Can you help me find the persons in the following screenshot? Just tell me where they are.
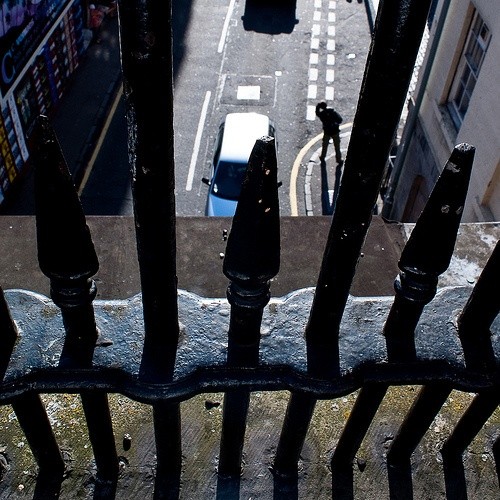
[94,5,117,62]
[316,101,343,164]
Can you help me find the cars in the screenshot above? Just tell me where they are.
[199,112,284,217]
